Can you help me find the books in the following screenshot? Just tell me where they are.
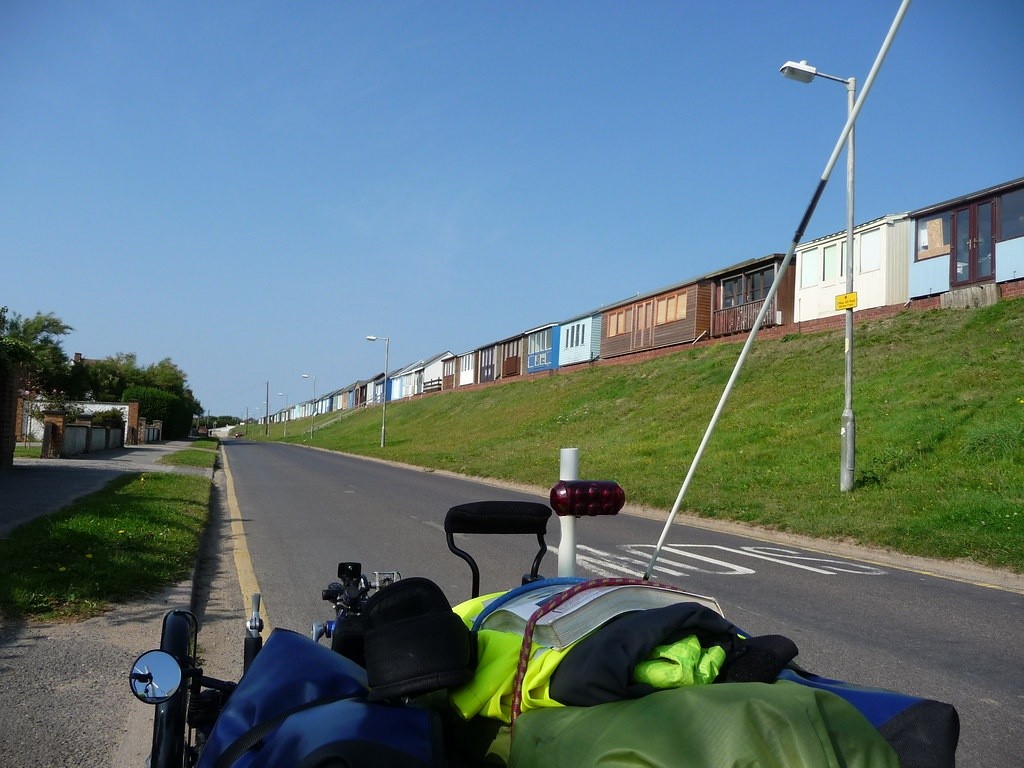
[468,584,725,651]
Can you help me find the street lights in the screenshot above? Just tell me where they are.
[779,58,859,490]
[277,392,288,437]
[365,335,390,448]
[255,407,263,436]
[302,374,316,439]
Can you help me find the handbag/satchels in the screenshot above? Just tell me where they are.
[198,627,430,768]
[361,577,468,700]
